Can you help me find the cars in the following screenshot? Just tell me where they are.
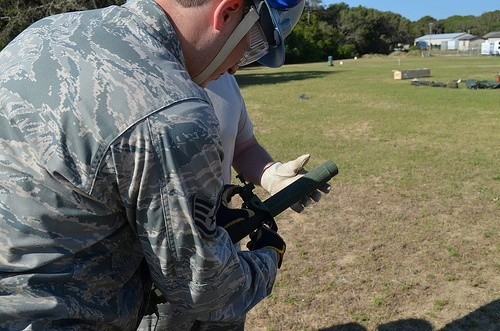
[393,46,405,51]
[488,81,500,89]
[432,82,447,88]
[403,44,411,51]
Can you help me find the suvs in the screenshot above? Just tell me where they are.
[466,79,478,89]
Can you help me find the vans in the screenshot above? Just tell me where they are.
[476,80,492,89]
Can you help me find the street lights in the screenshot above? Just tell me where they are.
[428,22,434,56]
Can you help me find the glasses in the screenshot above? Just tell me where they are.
[237,21,269,67]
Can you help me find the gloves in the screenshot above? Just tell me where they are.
[216,184,255,230]
[260,154,331,213]
[247,225,287,269]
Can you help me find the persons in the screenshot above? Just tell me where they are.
[137,70,331,331]
[0,0,305,331]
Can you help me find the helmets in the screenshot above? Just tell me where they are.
[250,0,305,69]
